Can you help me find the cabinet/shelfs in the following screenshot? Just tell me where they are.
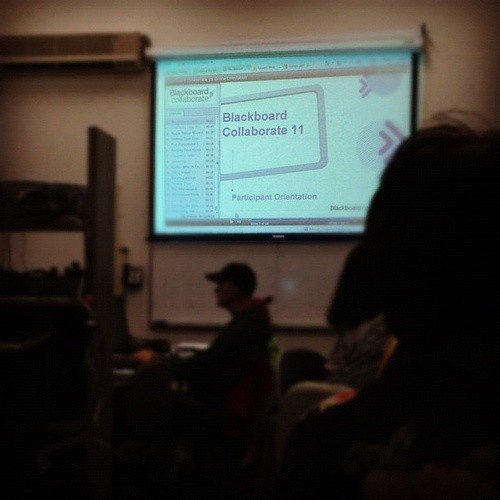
[0,122,117,500]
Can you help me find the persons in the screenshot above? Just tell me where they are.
[280,109,500,500]
[133,260,273,439]
[281,314,387,415]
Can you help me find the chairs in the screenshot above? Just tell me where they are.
[275,382,343,439]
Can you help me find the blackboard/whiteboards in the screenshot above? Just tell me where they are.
[147,234,360,337]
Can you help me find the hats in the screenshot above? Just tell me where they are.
[205,260,257,297]
